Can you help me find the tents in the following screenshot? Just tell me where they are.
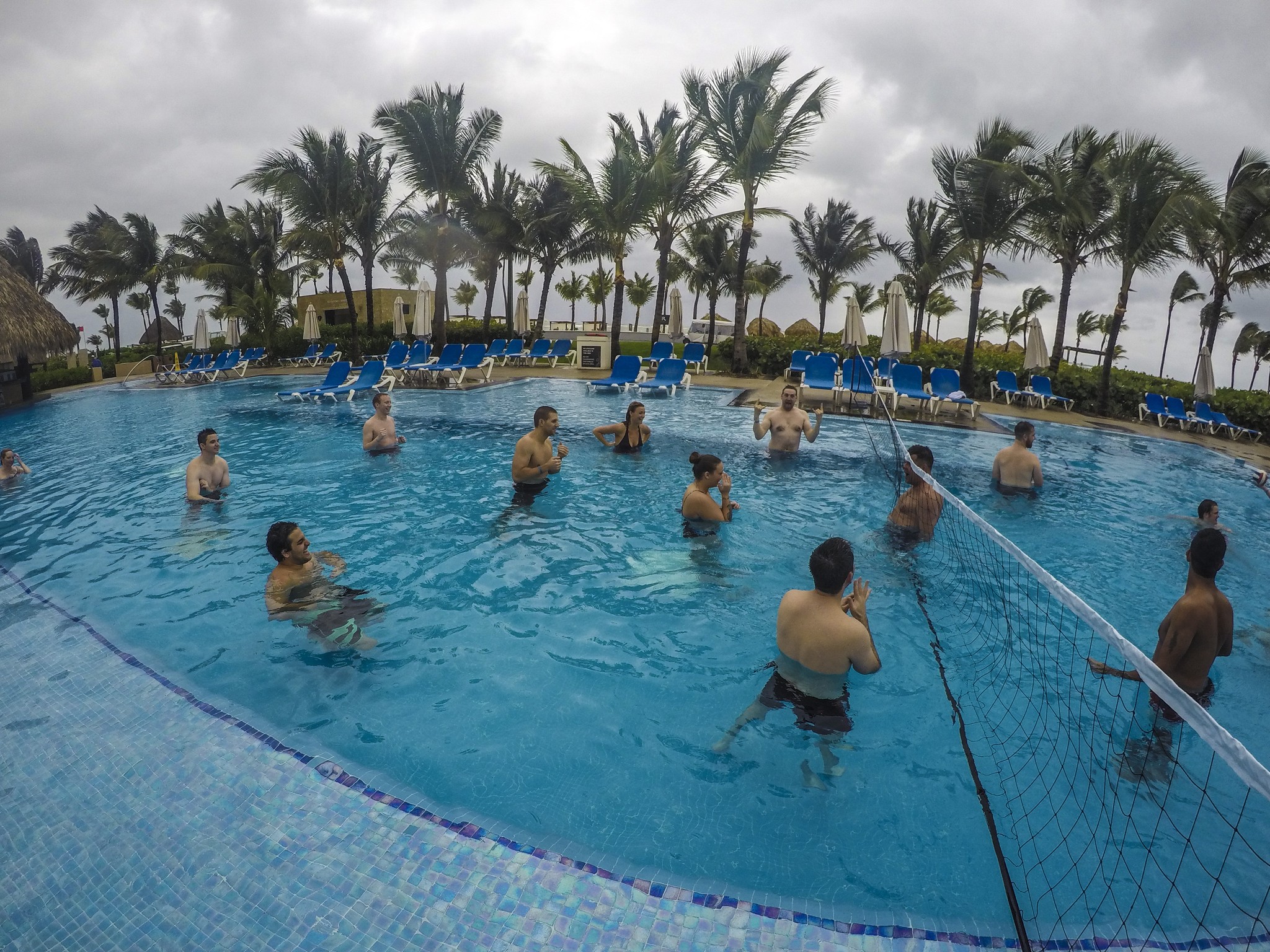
[701,312,1021,351]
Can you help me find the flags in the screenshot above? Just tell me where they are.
[77,326,83,332]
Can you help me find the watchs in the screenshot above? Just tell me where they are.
[753,419,759,423]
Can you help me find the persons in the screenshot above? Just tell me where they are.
[1088,499,1235,782]
[753,384,824,453]
[885,446,944,545]
[186,428,231,502]
[362,393,407,451]
[511,401,652,494]
[680,452,741,538]
[0,449,33,482]
[989,420,1047,488]
[1252,481,1270,498]
[265,520,378,652]
[712,538,881,754]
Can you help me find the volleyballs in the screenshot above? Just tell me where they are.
[1253,470,1267,486]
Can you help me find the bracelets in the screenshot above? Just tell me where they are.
[538,465,544,478]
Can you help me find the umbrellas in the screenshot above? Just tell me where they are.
[0,256,82,401]
[139,316,183,345]
[514,287,531,350]
[1193,346,1216,433]
[840,276,912,387]
[303,302,321,356]
[191,308,212,381]
[224,316,241,352]
[1021,312,1051,393]
[392,276,432,343]
[667,285,683,359]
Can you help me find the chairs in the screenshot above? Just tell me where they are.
[583,340,709,399]
[153,347,268,384]
[783,349,982,422]
[1138,391,1263,448]
[274,338,577,405]
[990,370,1076,414]
